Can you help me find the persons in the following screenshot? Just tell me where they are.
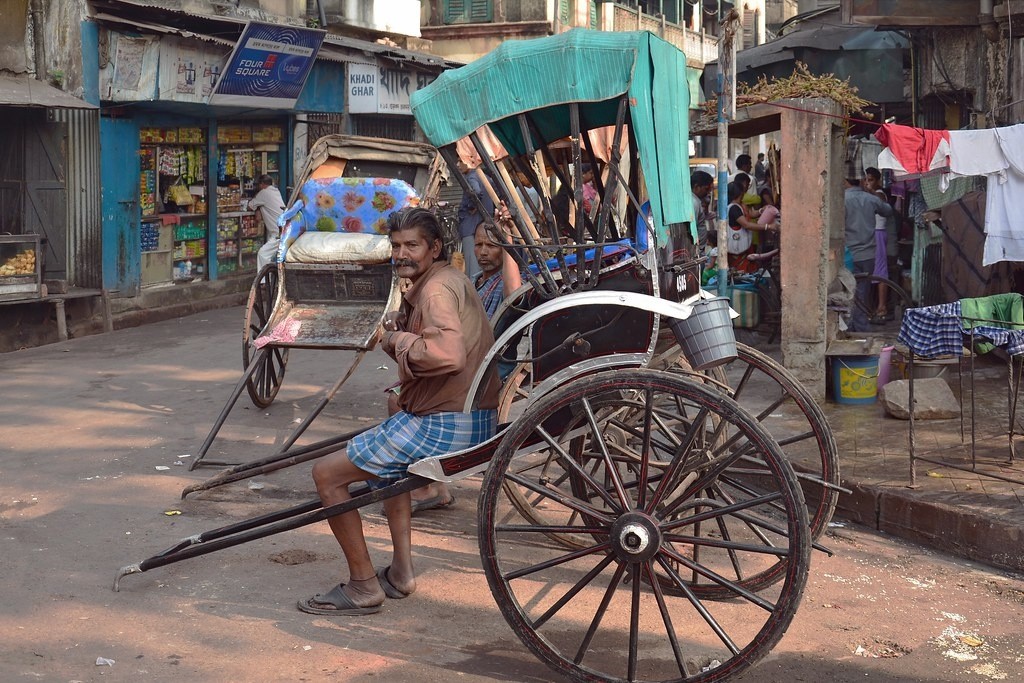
[247,175,286,291]
[379,153,902,516]
[297,208,506,615]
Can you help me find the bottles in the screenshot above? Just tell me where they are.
[173,242,187,259]
[178,259,192,277]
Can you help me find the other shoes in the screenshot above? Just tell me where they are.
[868,316,885,324]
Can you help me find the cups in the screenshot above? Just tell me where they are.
[757,205,779,225]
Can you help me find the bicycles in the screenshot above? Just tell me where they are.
[705,248,916,352]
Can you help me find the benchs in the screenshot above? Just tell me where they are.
[275,176,420,264]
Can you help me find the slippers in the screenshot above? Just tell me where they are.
[295,583,383,615]
[373,564,406,599]
[430,494,455,509]
[382,494,442,517]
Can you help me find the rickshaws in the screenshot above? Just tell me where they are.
[113,29,856,683]
[186,132,452,476]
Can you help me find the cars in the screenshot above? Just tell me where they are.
[688,157,735,199]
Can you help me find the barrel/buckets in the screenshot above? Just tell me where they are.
[664,269,739,371]
[856,339,895,393]
[912,364,951,385]
[828,354,880,405]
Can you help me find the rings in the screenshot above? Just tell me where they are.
[385,321,388,324]
[388,320,391,324]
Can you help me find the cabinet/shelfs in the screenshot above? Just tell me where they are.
[139,211,266,290]
